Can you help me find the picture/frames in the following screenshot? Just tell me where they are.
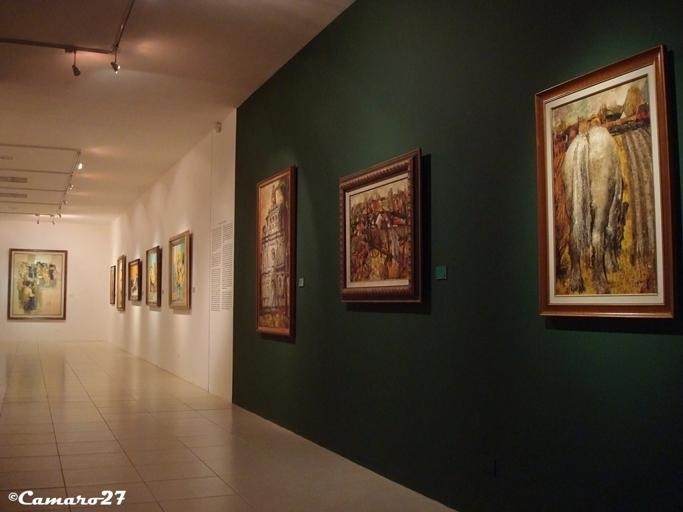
[7,248,68,321]
[255,165,297,337]
[167,229,193,310]
[534,44,676,322]
[115,254,126,310]
[337,146,425,306]
[109,266,116,305]
[146,246,162,307]
[128,258,142,301]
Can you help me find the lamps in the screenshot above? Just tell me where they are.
[109,52,121,75]
[69,51,82,80]
